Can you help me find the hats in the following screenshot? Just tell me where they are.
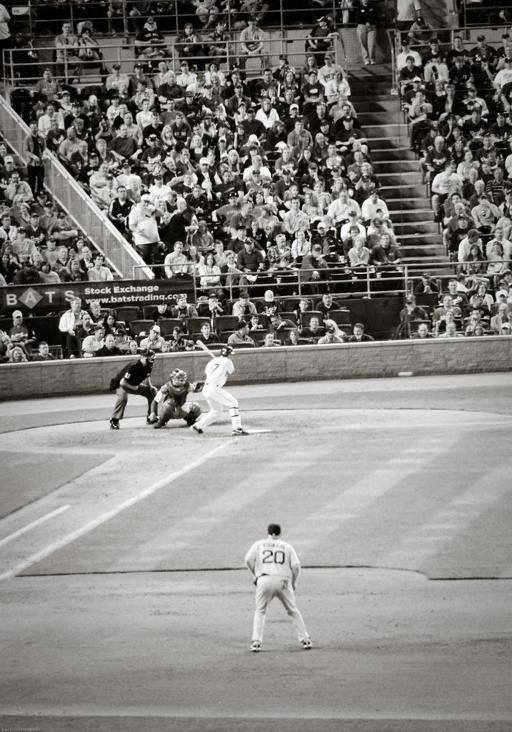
[497,280,508,286]
[423,272,431,278]
[207,289,274,303]
[151,326,161,334]
[269,525,280,536]
[405,294,416,304]
[401,34,509,265]
[13,310,22,318]
[5,7,390,269]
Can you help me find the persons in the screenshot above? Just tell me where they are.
[244,524,312,652]
[110,348,157,429]
[150,368,204,428]
[191,345,250,436]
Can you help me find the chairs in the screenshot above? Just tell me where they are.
[17,287,500,358]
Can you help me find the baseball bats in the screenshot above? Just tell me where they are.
[196,339,215,358]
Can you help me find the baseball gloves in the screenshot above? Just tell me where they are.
[192,381,204,392]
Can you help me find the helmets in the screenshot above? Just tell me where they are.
[170,368,186,392]
[221,346,235,356]
[140,348,155,369]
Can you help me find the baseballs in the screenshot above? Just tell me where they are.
[398,371,413,377]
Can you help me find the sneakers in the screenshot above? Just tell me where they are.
[303,640,312,649]
[154,421,166,428]
[147,415,158,424]
[111,421,120,429]
[187,421,197,427]
[232,429,248,435]
[191,425,203,433]
[249,643,261,651]
